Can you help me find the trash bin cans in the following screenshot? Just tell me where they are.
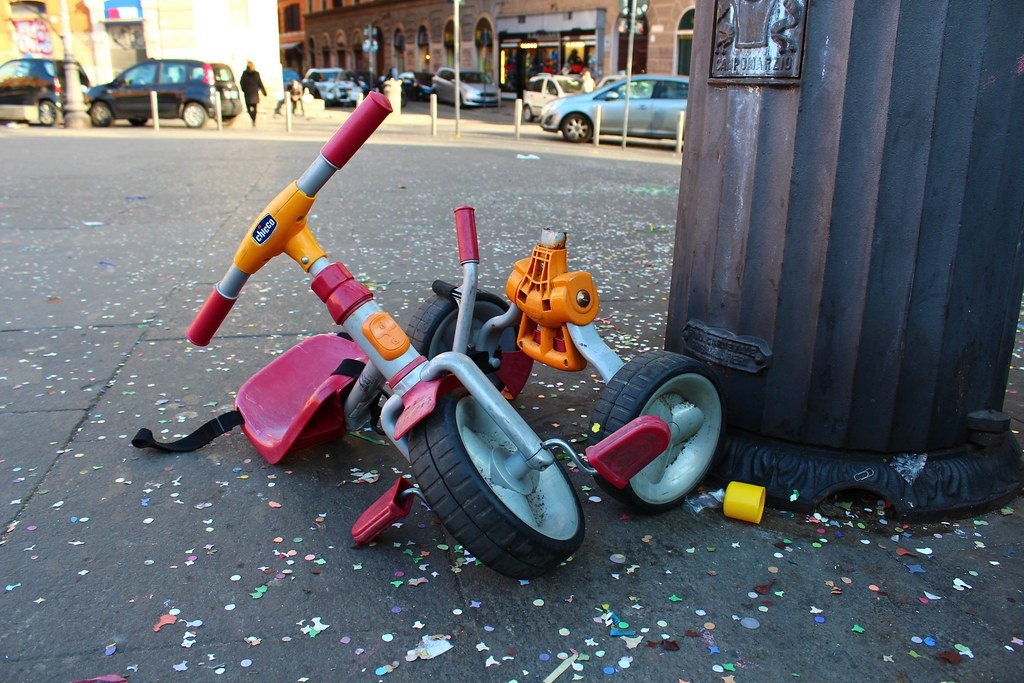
[659,1,1023,518]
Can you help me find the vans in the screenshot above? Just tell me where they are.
[432,67,499,106]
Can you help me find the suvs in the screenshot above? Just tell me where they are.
[83,58,242,129]
[521,72,584,122]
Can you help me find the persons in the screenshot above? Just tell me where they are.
[287,78,307,117]
[240,60,266,124]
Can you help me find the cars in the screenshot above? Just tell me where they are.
[282,69,300,89]
[0,57,94,128]
[332,72,405,111]
[539,75,689,144]
[303,67,348,102]
[400,72,438,101]
